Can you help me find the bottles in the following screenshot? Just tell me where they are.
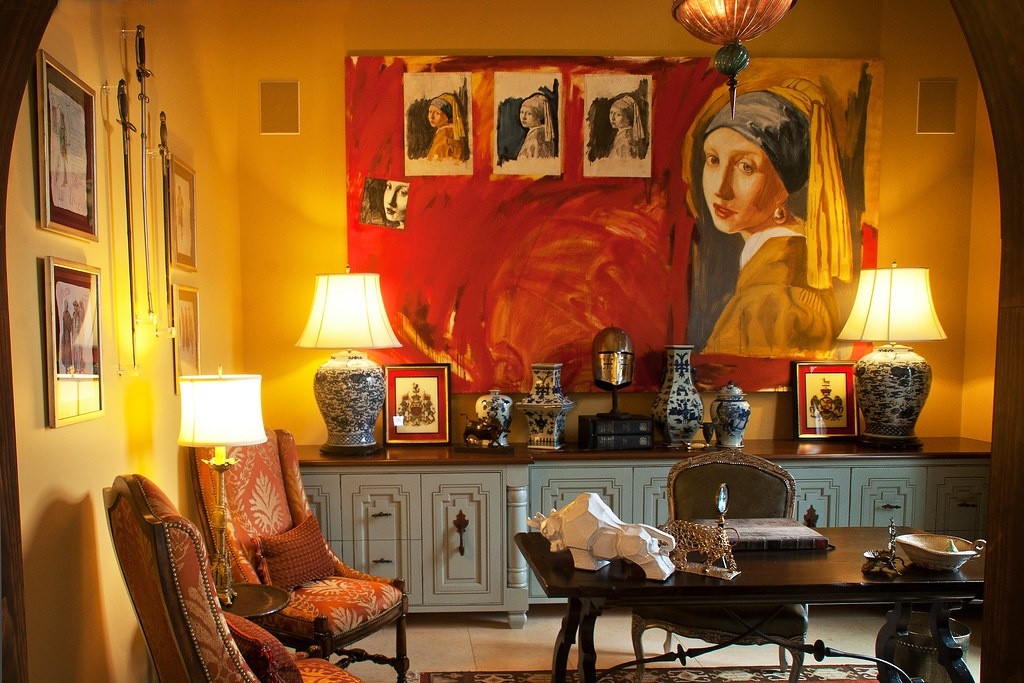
[652,344,704,450]
[710,381,751,448]
[475,390,513,447]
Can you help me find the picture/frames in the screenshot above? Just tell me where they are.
[35,50,200,429]
[382,365,453,449]
[792,361,859,443]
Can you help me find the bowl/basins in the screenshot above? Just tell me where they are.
[894,533,986,571]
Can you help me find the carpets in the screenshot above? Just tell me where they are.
[420,664,885,683]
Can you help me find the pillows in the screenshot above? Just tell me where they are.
[251,516,337,590]
[219,610,305,683]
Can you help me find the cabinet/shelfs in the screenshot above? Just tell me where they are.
[300,459,993,628]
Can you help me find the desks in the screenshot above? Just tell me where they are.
[216,584,292,618]
[513,525,985,683]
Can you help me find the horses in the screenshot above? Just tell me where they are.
[463,419,511,449]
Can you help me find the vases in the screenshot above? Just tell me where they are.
[476,362,574,450]
[653,345,704,449]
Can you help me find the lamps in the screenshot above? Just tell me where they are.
[178,375,268,605]
[672,0,799,118]
[835,268,948,451]
[296,274,403,456]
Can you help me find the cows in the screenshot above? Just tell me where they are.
[654,519,741,575]
[525,492,676,583]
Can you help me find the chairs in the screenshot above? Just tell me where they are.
[103,429,409,683]
[632,450,808,682]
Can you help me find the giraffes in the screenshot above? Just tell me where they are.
[861,518,903,576]
